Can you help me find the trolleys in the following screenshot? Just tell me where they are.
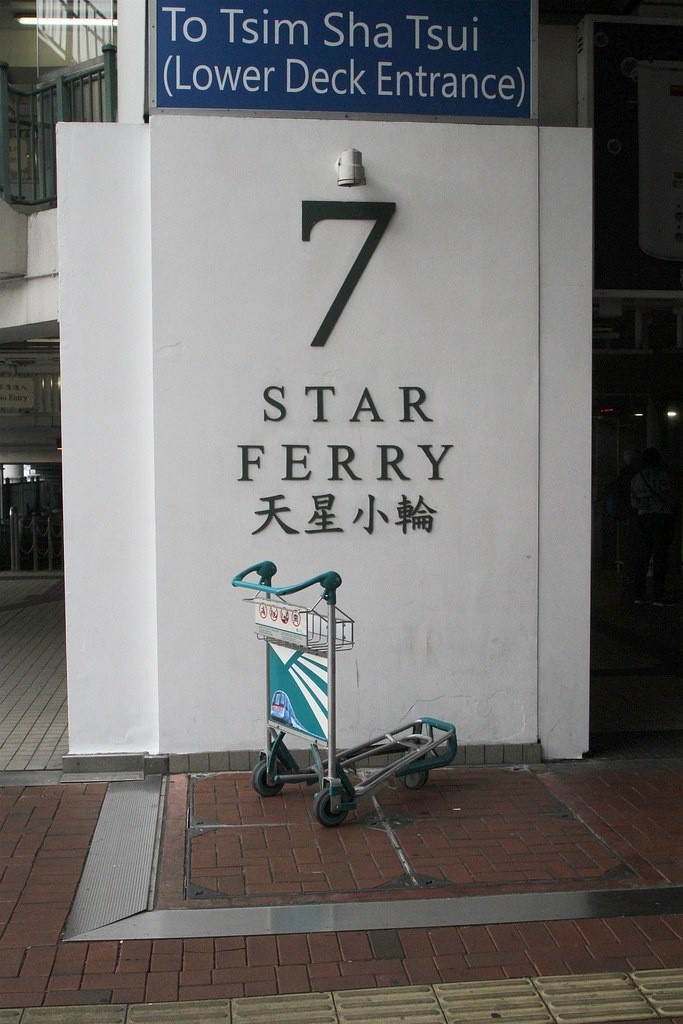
[231,560,457,828]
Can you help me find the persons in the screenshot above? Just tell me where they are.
[618,445,642,598]
[628,449,676,607]
